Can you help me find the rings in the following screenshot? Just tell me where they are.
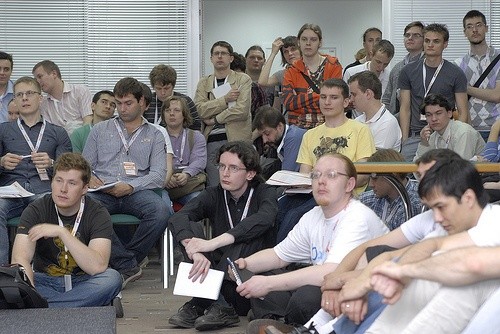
[346,305,350,308]
[325,302,329,304]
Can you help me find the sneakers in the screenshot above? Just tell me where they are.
[169,297,205,328]
[264,320,320,334]
[195,303,240,330]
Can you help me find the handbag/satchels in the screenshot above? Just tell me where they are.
[168,168,207,199]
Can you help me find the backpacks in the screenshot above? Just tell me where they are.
[0,263,48,309]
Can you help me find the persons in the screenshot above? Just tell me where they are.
[10,153,124,318]
[227,151,390,325]
[246,148,500,334]
[167,141,278,330]
[0,10,500,287]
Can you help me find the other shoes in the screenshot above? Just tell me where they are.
[137,256,150,269]
[120,265,143,289]
[110,298,123,318]
[246,320,296,334]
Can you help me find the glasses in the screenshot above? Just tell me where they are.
[310,171,349,181]
[464,23,486,29]
[213,51,231,57]
[14,90,41,98]
[217,163,247,173]
[403,33,424,39]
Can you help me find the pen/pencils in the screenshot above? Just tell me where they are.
[426,123,430,143]
[20,154,31,158]
[93,171,105,186]
[227,258,235,267]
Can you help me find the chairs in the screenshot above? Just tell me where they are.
[73,127,175,292]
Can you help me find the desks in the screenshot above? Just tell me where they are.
[355,163,500,173]
